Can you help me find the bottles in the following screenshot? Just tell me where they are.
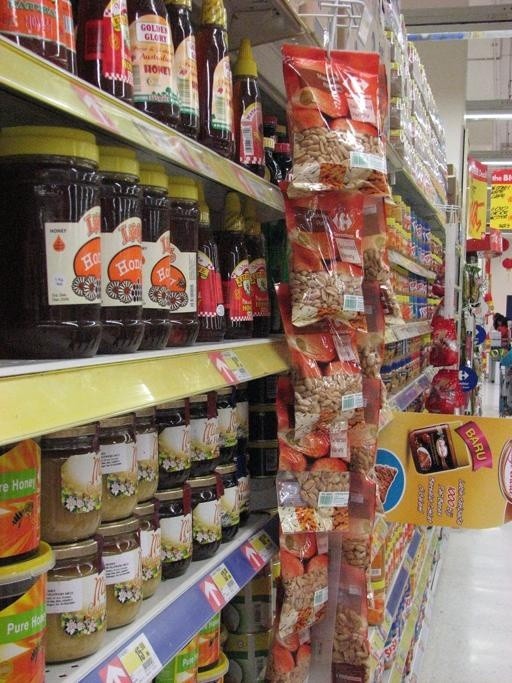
[1,1,289,186]
[195,180,272,343]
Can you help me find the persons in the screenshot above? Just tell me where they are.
[493,313,508,330]
[496,315,508,338]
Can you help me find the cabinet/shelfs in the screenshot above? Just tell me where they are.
[0,0,486,683]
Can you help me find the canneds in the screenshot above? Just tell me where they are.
[0,126,278,683]
